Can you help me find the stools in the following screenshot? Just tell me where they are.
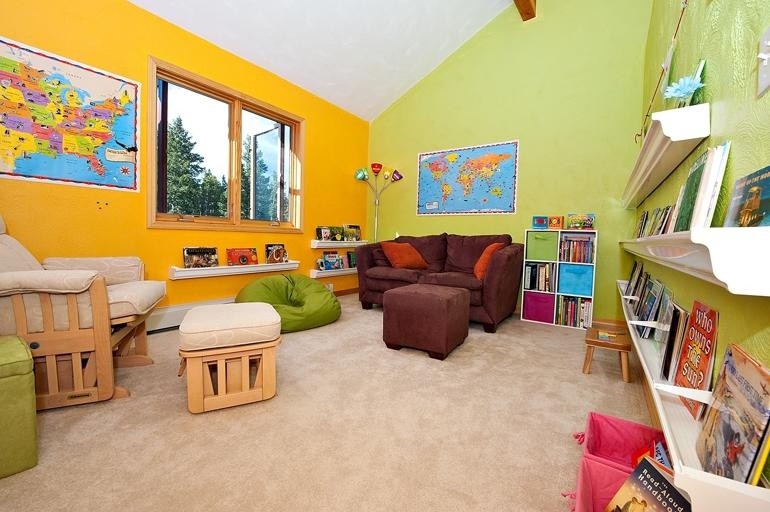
[582,318,632,384]
[0,333,39,481]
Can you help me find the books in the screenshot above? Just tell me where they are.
[525,261,557,292]
[652,286,719,422]
[315,223,362,270]
[623,259,664,339]
[695,342,770,485]
[605,439,692,512]
[723,165,770,227]
[669,140,731,232]
[631,204,675,239]
[559,236,594,263]
[554,293,591,331]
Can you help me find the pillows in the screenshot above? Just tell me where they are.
[472,242,505,282]
[379,241,430,271]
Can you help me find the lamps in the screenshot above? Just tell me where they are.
[354,159,404,243]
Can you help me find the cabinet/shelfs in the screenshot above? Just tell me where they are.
[615,100,770,511]
[306,236,359,279]
[520,228,599,331]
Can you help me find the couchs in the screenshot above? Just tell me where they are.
[235,273,342,335]
[382,282,472,361]
[177,300,282,417]
[0,209,168,413]
[354,231,524,334]
